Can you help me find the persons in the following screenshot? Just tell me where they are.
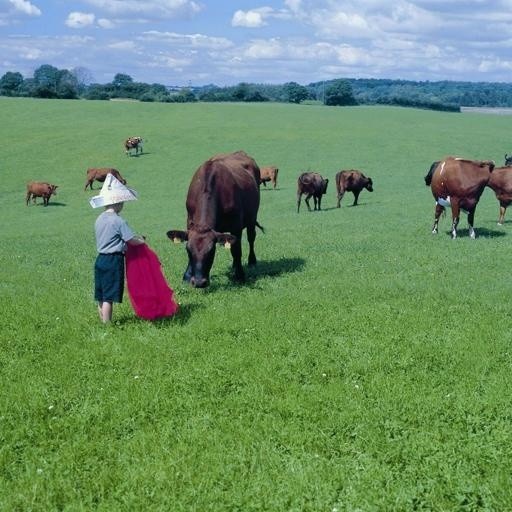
[90,172,138,324]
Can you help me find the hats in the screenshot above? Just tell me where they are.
[90,173,138,209]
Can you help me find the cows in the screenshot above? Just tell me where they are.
[334,169,374,208]
[83,167,128,192]
[125,136,144,156]
[25,181,59,207]
[259,165,279,190]
[424,155,496,241]
[295,171,329,214]
[485,164,512,226]
[165,151,266,289]
